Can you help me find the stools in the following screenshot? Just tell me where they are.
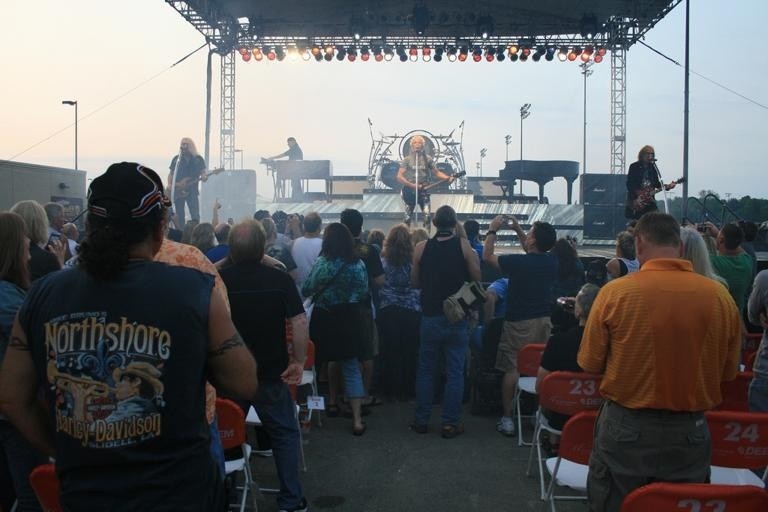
[493,181,516,204]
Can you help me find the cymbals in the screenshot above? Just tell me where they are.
[432,136,450,138]
[385,136,403,137]
[445,143,460,146]
[379,154,392,156]
[375,141,390,144]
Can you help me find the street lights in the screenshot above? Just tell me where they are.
[516,102,534,203]
[475,161,480,177]
[480,148,487,178]
[579,58,594,173]
[503,134,512,162]
[60,97,80,174]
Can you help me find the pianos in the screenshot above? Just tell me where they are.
[499,160,579,205]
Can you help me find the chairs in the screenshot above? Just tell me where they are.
[10,333,323,512]
[510,333,768,512]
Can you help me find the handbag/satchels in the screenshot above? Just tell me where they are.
[443,281,486,324]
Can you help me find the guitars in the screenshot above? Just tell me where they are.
[632,176,686,211]
[402,170,466,205]
[166,166,225,204]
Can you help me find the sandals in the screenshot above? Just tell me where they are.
[325,395,382,436]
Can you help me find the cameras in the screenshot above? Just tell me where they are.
[500,216,509,227]
[557,297,575,310]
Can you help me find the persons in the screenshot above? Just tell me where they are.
[267,136,308,198]
[623,145,677,220]
[538,211,768,512]
[397,136,456,229]
[1,203,86,511]
[378,205,584,439]
[164,137,208,232]
[164,205,387,512]
[0,161,260,505]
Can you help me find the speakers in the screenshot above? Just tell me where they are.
[583,173,628,206]
[584,206,626,240]
[201,170,257,229]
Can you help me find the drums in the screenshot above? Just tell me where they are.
[399,130,439,167]
[433,163,454,186]
[381,163,404,187]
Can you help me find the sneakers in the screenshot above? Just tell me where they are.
[440,424,460,437]
[280,497,308,512]
[409,422,428,433]
[496,417,514,436]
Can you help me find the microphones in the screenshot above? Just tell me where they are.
[415,146,419,152]
[648,158,657,161]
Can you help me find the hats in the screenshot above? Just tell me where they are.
[88,163,171,226]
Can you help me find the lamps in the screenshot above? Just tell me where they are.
[237,0,606,63]
